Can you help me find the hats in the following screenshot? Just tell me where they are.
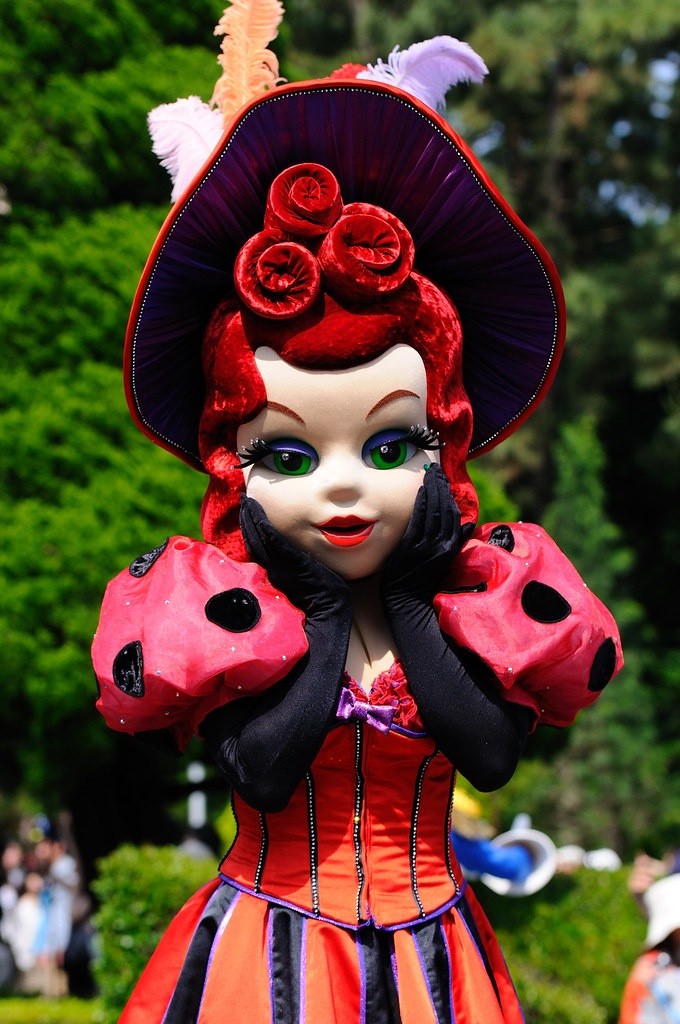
[642,873,680,950]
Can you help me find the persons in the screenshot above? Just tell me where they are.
[0,823,100,1001]
[618,822,680,1024]
[90,77,626,1024]
